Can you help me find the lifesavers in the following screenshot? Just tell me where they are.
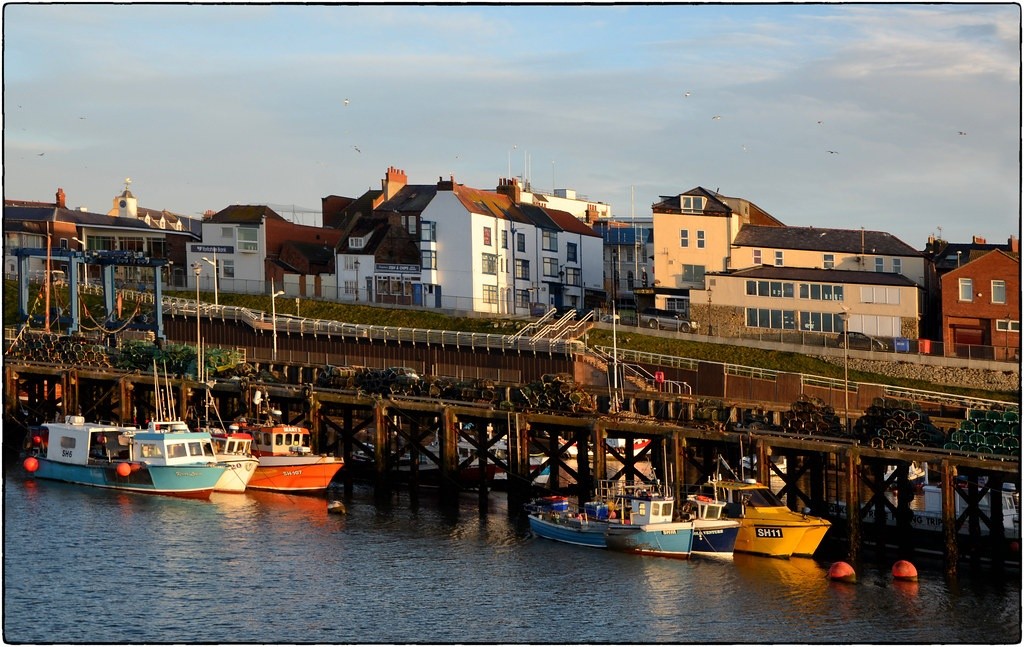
[695,496,712,502]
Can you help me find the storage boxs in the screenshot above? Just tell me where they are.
[585,502,608,519]
[543,496,569,510]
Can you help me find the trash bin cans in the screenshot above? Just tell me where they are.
[893,337,909,353]
[917,338,931,355]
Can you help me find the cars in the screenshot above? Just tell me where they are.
[836,331,888,352]
[600,314,617,324]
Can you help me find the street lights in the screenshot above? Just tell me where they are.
[706,286,714,336]
[201,247,218,314]
[270,276,285,361]
[837,312,852,438]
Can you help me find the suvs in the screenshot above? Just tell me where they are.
[638,307,702,333]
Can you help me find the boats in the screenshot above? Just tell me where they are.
[191,367,345,495]
[18,357,229,501]
[523,477,742,563]
[352,416,555,496]
[676,471,833,557]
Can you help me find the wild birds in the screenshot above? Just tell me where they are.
[956,131,966,135]
[354,146,361,153]
[828,151,838,154]
[684,91,693,97]
[344,99,349,106]
[818,121,823,124]
[36,152,44,156]
[711,115,721,120]
